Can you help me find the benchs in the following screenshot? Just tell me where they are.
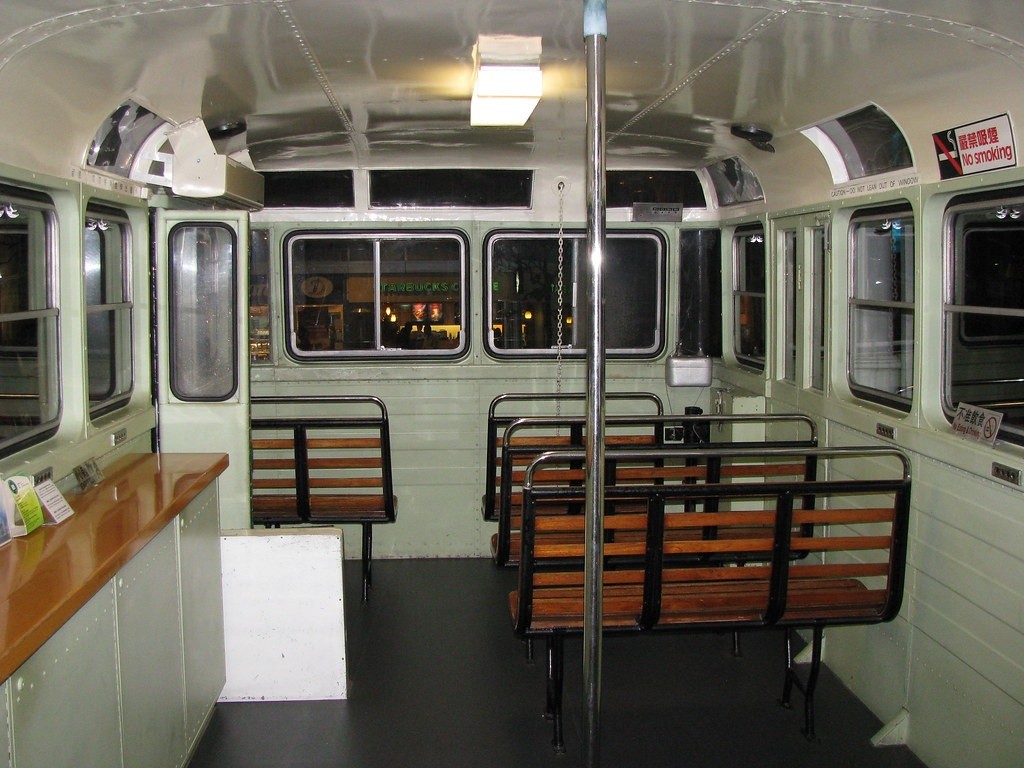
[480,391,663,521]
[489,413,817,663]
[249,395,397,603]
[509,445,912,757]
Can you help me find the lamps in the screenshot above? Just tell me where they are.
[471,33,542,71]
[470,66,543,126]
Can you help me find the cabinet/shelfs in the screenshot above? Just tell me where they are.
[0,453,229,768]
